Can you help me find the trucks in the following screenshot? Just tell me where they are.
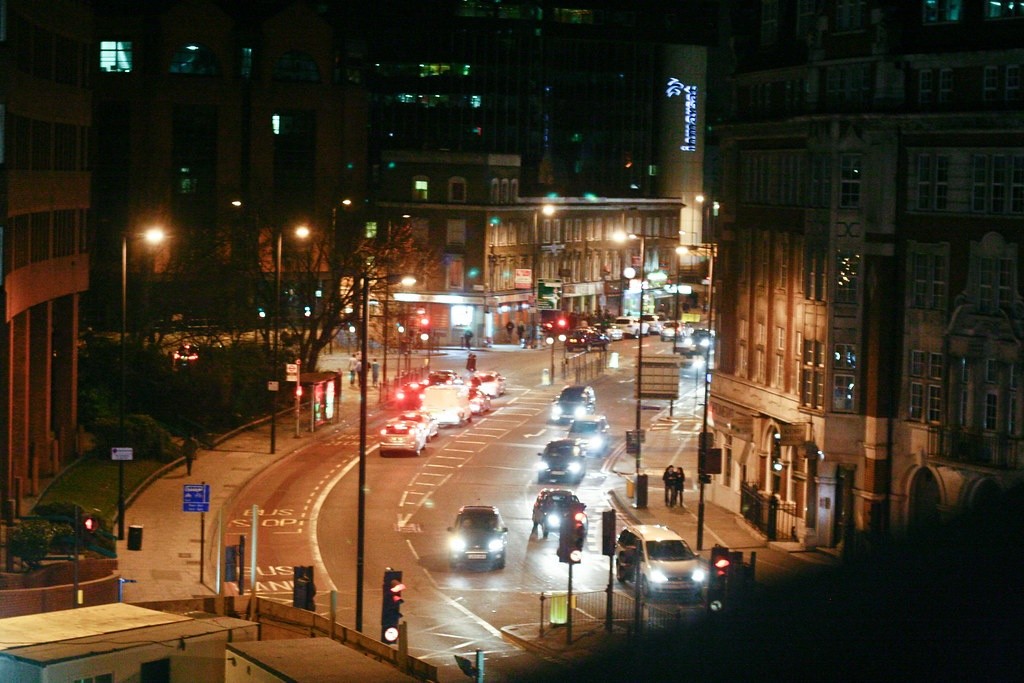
[423,385,472,427]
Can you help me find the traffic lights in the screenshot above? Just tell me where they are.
[382,570,405,645]
[706,548,730,613]
[565,502,588,551]
[83,517,97,539]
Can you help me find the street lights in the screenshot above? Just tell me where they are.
[672,241,715,551]
[615,233,643,470]
[118,229,168,538]
[271,223,307,450]
[533,206,556,345]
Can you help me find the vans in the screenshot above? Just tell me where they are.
[568,415,611,457]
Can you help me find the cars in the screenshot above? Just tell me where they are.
[538,439,588,485]
[565,323,622,352]
[398,370,505,416]
[379,411,438,456]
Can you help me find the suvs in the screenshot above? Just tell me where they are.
[615,313,712,355]
[533,489,579,539]
[614,526,704,601]
[551,384,597,423]
[450,506,508,571]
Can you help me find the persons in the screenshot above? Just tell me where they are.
[465,320,534,371]
[673,467,685,506]
[663,465,675,507]
[184,433,198,475]
[348,354,380,386]
